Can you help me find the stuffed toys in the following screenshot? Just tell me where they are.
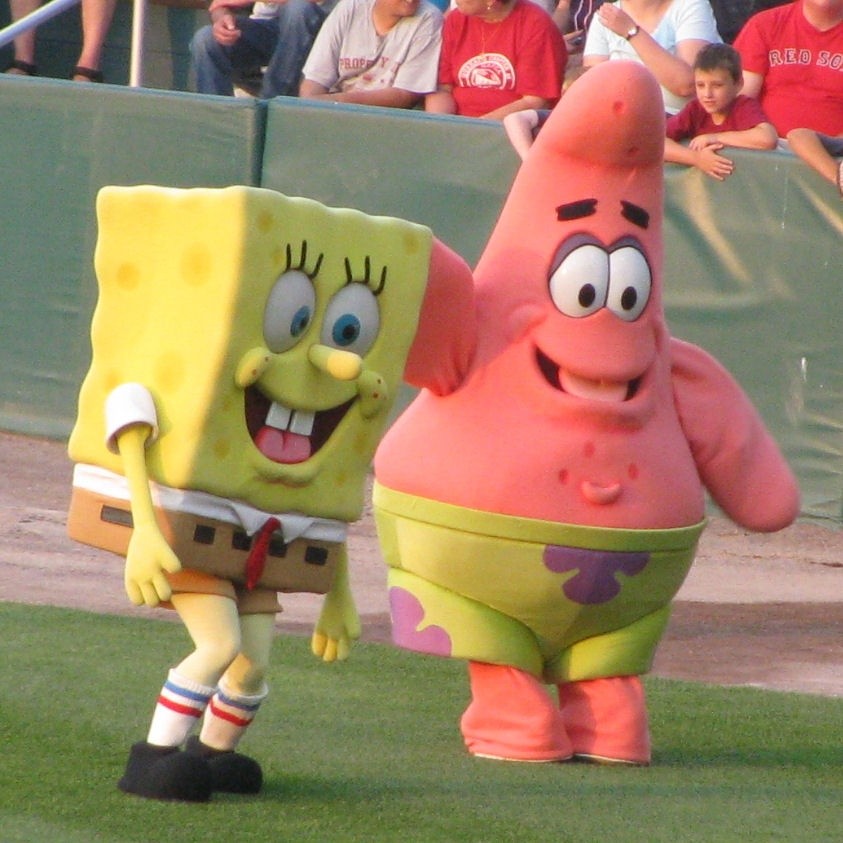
[373,59,801,767]
[64,185,433,803]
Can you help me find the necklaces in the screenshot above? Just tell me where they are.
[479,7,511,58]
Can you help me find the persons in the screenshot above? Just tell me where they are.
[4,0,843,197]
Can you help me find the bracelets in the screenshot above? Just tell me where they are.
[627,26,640,41]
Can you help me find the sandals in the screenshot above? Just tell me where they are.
[68,65,103,83]
[1,59,39,77]
